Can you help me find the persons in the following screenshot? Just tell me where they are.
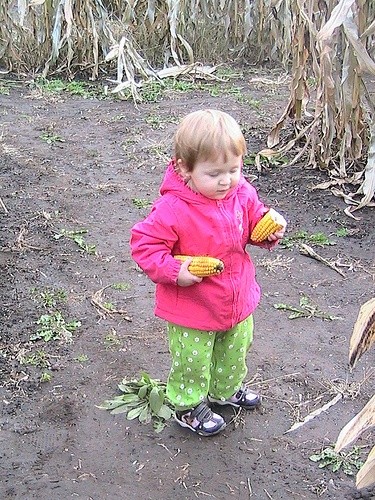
[128,110,289,436]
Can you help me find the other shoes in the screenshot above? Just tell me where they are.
[173,401,226,437]
[208,384,259,410]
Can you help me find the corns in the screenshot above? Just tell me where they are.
[174,254,224,277]
[349,297,375,371]
[251,208,283,243]
